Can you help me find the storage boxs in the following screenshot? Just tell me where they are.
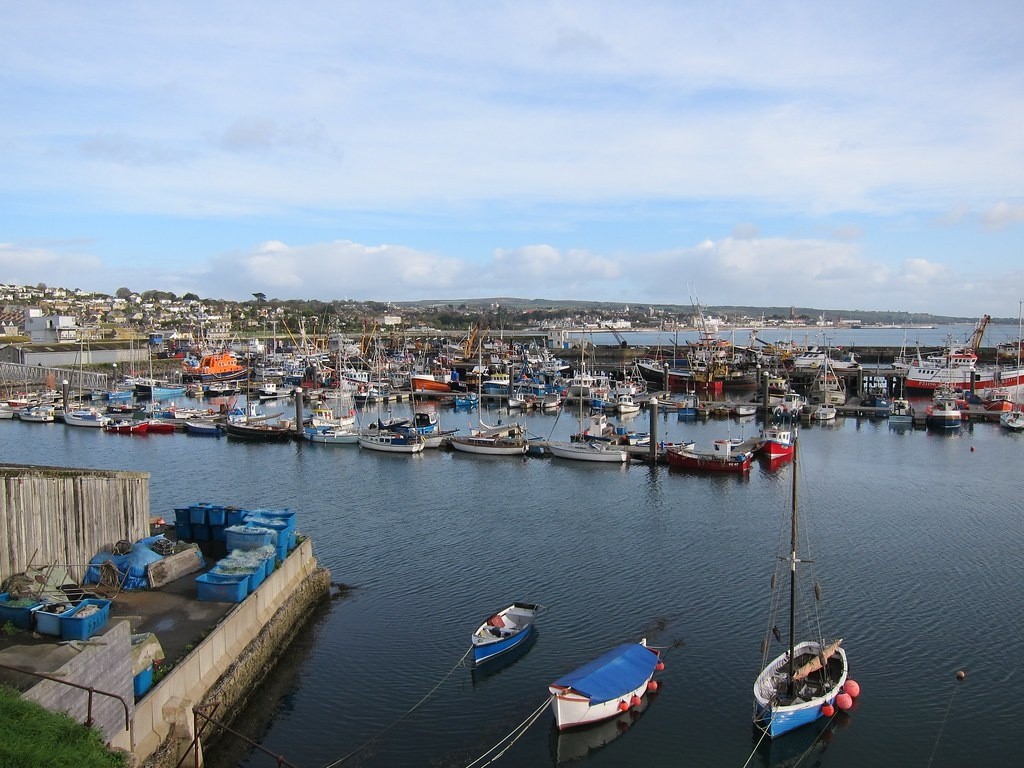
[616,426,627,435]
[172,502,295,603]
[135,663,153,695]
[0,592,112,641]
[235,410,244,415]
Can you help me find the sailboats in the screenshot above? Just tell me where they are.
[113,333,169,386]
[227,345,293,440]
[64,332,111,426]
[359,334,425,452]
[451,336,529,454]
[754,428,860,738]
[548,323,631,461]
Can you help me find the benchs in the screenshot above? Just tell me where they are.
[483,625,518,635]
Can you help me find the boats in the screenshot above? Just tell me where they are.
[889,377,915,423]
[754,700,860,768]
[0,384,695,462]
[666,439,751,474]
[397,402,441,434]
[471,602,538,665]
[927,387,961,428]
[891,314,1024,411]
[182,316,860,420]
[549,637,665,731]
[570,411,650,446]
[1000,411,1024,428]
[755,423,795,456]
[472,625,539,688]
[549,691,653,768]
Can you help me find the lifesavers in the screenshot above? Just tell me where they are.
[208,407,213,413]
[420,436,426,442]
[325,414,331,419]
